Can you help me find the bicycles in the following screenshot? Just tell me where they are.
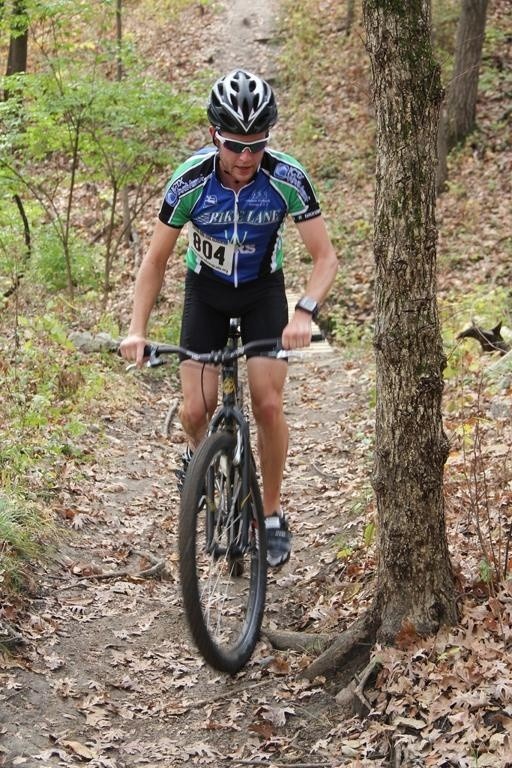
[118,305,324,677]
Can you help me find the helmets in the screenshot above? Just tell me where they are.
[207,68,278,133]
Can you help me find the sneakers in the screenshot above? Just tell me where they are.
[262,506,291,570]
[176,444,206,513]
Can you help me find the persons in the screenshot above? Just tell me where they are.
[117,65,342,571]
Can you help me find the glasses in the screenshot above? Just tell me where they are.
[215,136,272,155]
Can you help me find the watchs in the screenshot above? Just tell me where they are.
[295,296,319,318]
